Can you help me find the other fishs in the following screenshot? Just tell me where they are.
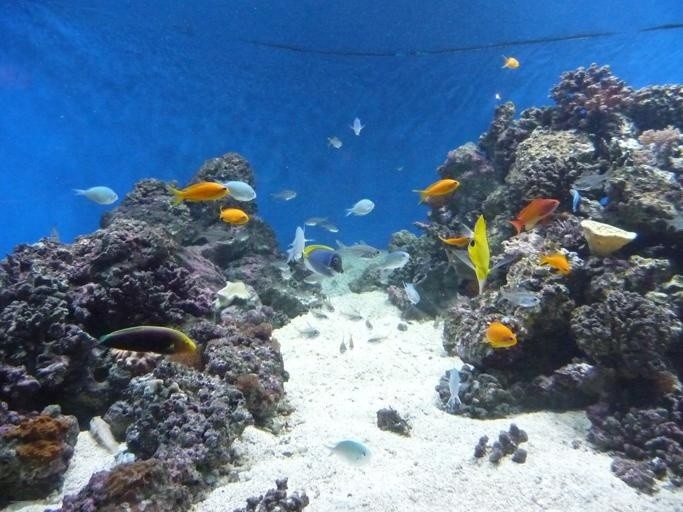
[268,164,615,355]
